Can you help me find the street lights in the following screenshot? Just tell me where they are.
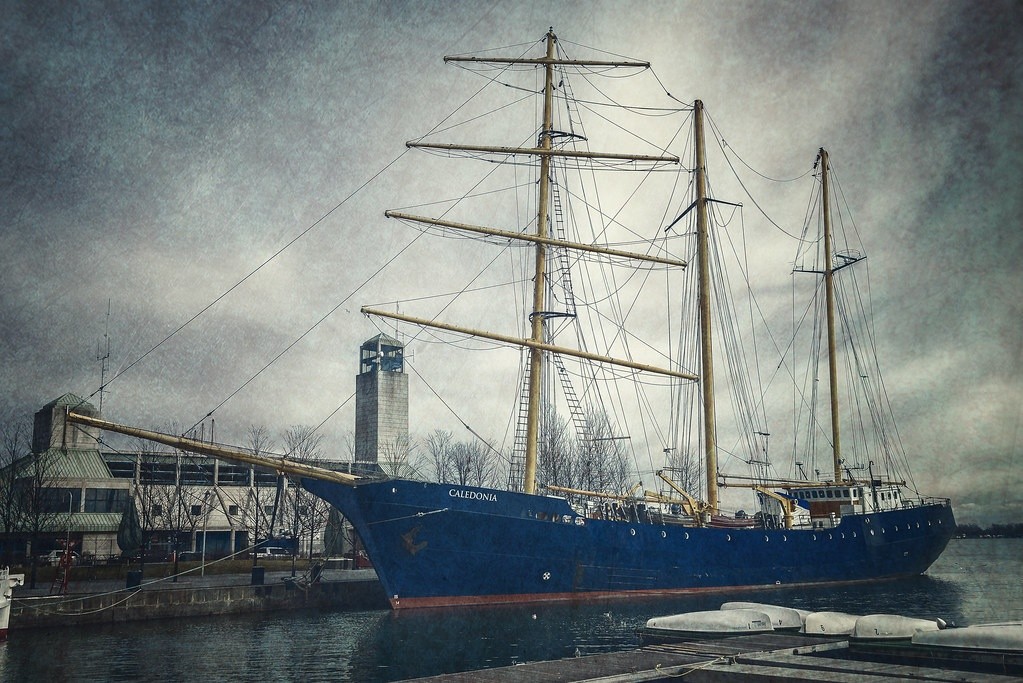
[62,491,74,580]
[202,490,217,577]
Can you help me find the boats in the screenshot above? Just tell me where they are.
[648,512,697,526]
[646,601,1023,650]
[705,516,755,528]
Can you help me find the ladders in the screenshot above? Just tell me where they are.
[50,546,73,595]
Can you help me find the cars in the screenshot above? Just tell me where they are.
[36,549,82,568]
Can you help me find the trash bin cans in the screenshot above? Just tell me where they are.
[126,570,143,591]
[252,566,265,585]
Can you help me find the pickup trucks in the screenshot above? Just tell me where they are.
[247,546,290,558]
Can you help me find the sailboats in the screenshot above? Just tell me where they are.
[66,32,962,612]
